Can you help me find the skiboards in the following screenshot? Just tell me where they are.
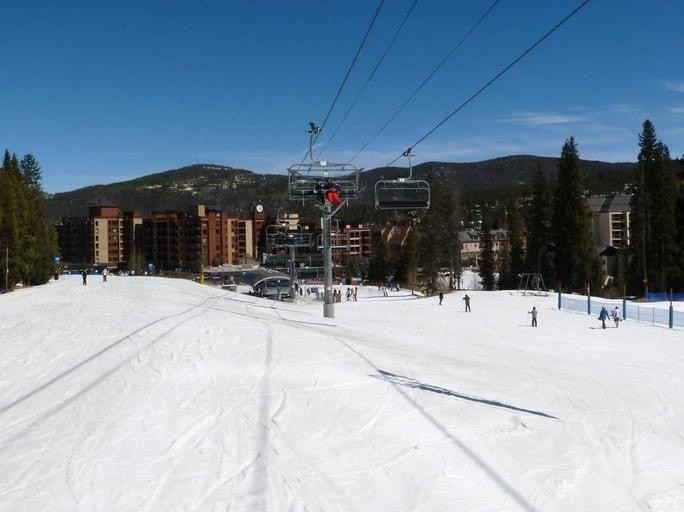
[312,203,331,218]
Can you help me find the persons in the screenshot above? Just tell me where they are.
[312,179,324,205]
[463,294,471,312]
[101,267,109,283]
[611,306,622,328]
[528,307,538,327]
[81,269,87,285]
[599,306,610,329]
[438,291,443,305]
[298,286,358,302]
[324,176,341,205]
